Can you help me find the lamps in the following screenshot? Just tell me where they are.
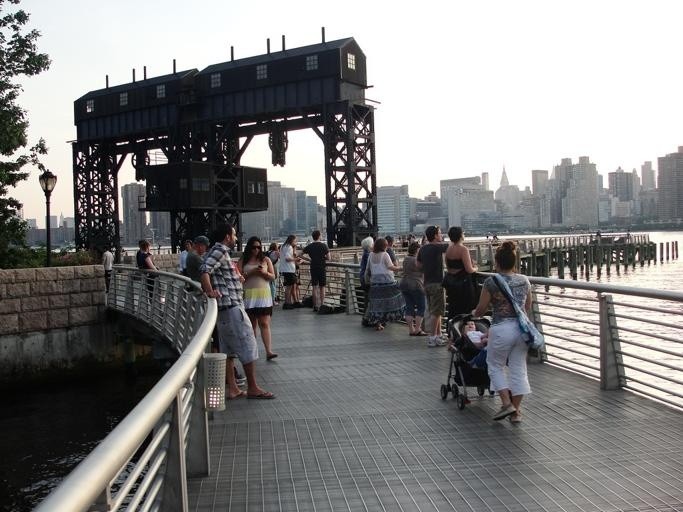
[204,354,226,411]
[160,283,167,303]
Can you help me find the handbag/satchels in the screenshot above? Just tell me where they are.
[518,313,544,350]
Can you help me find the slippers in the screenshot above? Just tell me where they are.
[227,390,247,400]
[246,391,279,399]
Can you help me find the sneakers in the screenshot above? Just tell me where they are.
[491,402,523,423]
[410,331,450,346]
[226,374,245,386]
[266,353,277,361]
[282,303,293,309]
[294,302,304,308]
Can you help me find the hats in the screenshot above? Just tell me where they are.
[194,235,210,246]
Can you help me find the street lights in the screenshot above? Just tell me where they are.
[38,169,58,266]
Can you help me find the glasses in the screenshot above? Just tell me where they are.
[251,246,261,250]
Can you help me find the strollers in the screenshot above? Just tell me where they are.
[441,314,495,411]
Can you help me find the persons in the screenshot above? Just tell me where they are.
[359,225,479,348]
[585,226,634,248]
[238,237,277,360]
[102,245,113,294]
[199,224,275,401]
[266,230,331,311]
[136,239,160,305]
[468,237,536,426]
[177,236,247,387]
